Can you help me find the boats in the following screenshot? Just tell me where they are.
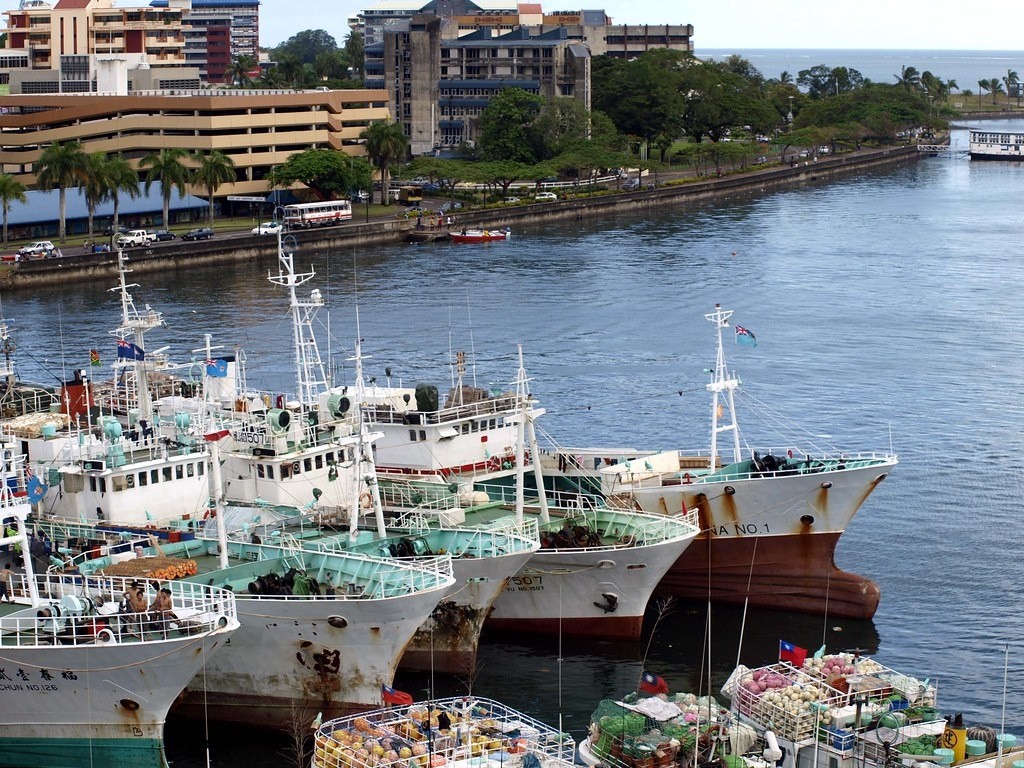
[967,128,1024,162]
[449,227,513,245]
[0,224,1024,768]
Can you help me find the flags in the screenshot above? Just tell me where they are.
[734,324,755,343]
[778,639,808,669]
[381,683,413,710]
[25,462,48,503]
[89,348,102,366]
[116,338,144,363]
[641,670,669,695]
[204,357,228,376]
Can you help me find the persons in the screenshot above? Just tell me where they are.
[717,168,723,179]
[562,191,568,200]
[647,182,655,190]
[403,208,468,236]
[82,239,112,256]
[483,228,489,237]
[149,580,181,638]
[14,244,64,263]
[123,581,149,641]
[140,237,152,248]
[0,563,15,604]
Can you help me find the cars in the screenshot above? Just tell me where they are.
[715,132,829,166]
[247,182,463,236]
[495,167,654,207]
[1,221,214,263]
